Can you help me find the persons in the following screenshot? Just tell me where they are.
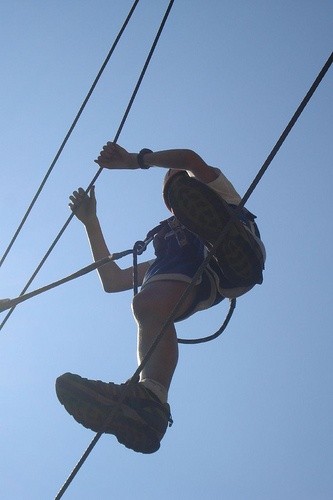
[55,142,266,455]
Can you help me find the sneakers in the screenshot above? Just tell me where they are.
[166,178,265,287]
[55,371,171,454]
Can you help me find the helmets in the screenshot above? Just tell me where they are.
[162,167,187,214]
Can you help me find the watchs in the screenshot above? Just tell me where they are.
[138,147,154,169]
